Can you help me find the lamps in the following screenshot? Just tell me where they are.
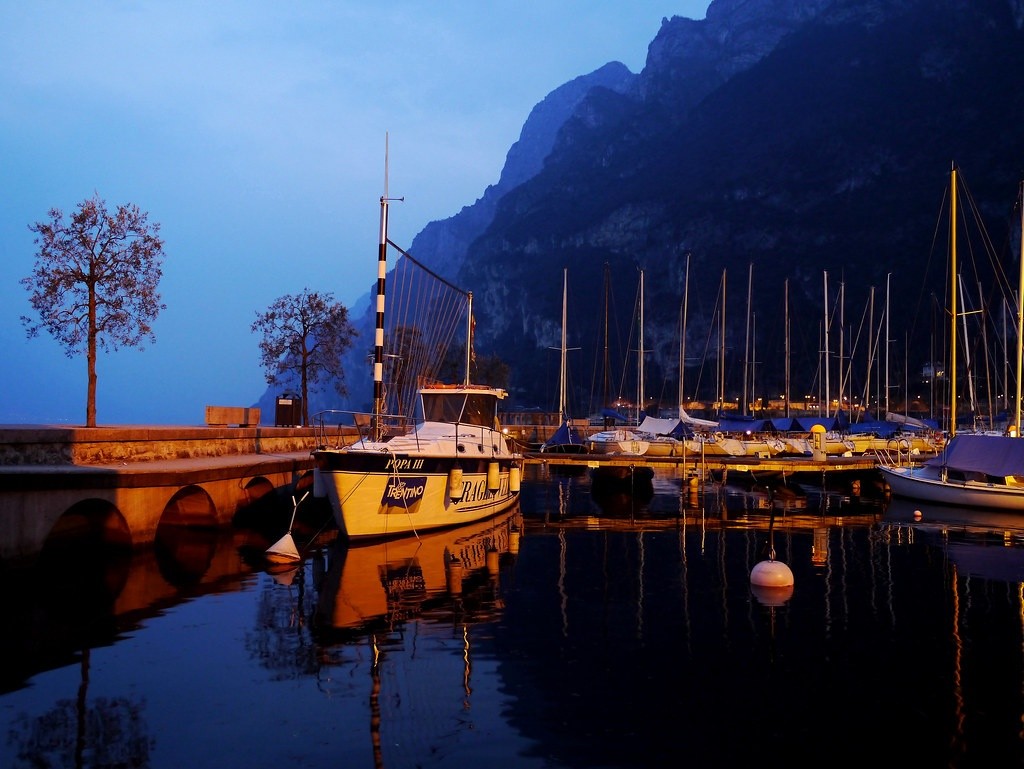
[510,462,520,496]
[590,442,594,451]
[450,459,463,506]
[488,456,501,497]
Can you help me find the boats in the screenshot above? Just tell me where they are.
[306,502,527,769]
[309,132,527,546]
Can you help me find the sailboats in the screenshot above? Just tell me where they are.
[875,218,1024,510]
[541,170,1024,457]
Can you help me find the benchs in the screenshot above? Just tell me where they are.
[207,406,261,428]
[354,414,372,427]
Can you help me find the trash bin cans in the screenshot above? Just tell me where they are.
[273,389,303,427]
